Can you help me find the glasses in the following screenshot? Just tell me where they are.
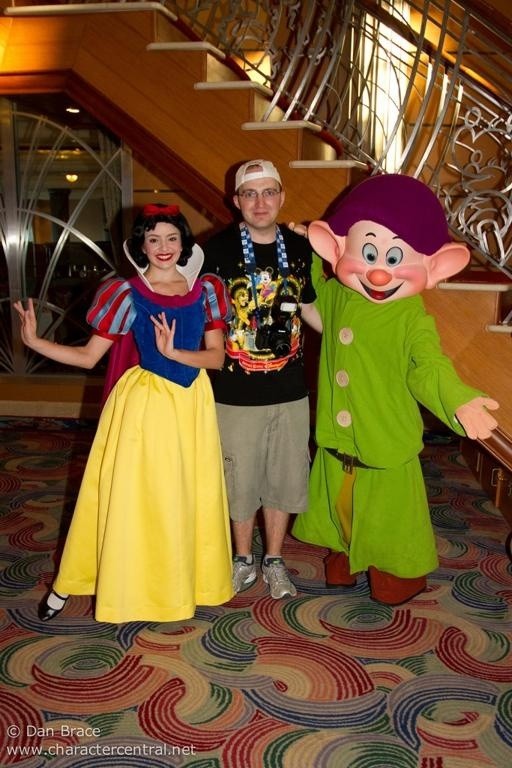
[237,188,281,200]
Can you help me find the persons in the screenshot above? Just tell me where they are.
[14,204,237,624]
[197,160,322,597]
[287,173,500,605]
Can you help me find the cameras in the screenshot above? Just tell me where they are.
[255,321,291,358]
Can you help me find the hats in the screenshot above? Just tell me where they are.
[234,159,283,191]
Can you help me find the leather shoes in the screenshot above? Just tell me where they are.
[39,590,69,622]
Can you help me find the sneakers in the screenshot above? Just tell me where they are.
[232,553,257,594]
[261,558,298,600]
